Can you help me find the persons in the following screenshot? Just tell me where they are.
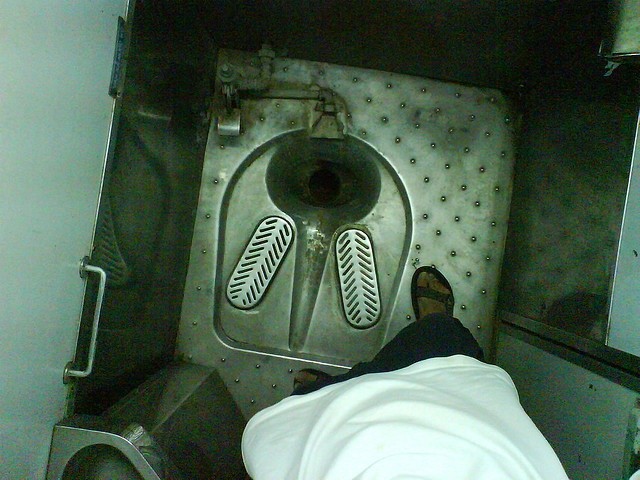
[238,265,570,480]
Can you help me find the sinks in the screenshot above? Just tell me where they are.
[44,429,148,479]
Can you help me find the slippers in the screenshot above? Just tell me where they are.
[294,368,333,387]
[411,266,455,321]
